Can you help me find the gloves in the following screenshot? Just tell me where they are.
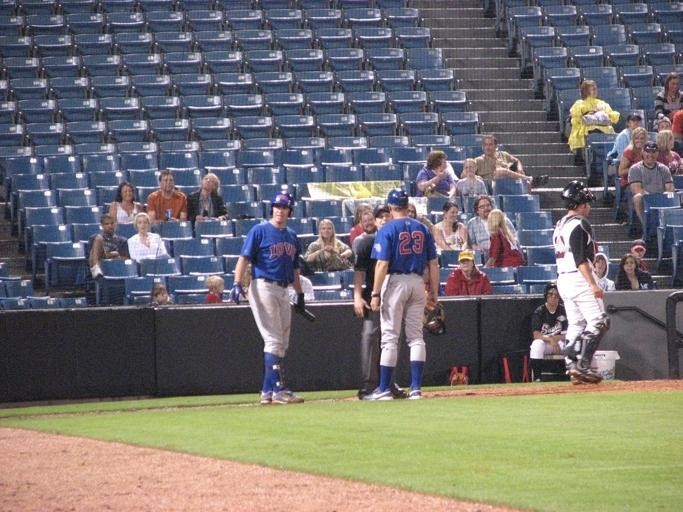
[229,282,246,305]
[293,292,305,314]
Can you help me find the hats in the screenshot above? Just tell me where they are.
[627,112,641,121]
[642,142,659,152]
[630,239,648,253]
[372,204,390,217]
[458,251,475,262]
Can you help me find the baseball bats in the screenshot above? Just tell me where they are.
[290,302,315,321]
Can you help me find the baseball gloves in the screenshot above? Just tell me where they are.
[425,302,445,335]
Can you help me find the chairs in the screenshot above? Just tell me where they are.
[483,0,683,290]
[1,0,621,308]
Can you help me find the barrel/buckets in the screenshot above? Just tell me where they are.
[590,350,621,379]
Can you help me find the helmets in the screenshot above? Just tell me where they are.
[388,188,408,207]
[268,191,295,217]
[560,180,596,210]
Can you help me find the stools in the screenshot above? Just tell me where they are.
[531,354,565,382]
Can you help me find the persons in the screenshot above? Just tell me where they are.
[362,188,439,401]
[553,180,610,385]
[89,171,231,306]
[568,72,683,221]
[594,239,655,290]
[529,284,568,383]
[229,191,306,404]
[353,205,408,400]
[289,209,375,303]
[407,135,532,295]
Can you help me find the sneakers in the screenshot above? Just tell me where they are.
[357,381,422,401]
[90,265,103,279]
[567,359,603,385]
[259,390,305,404]
[531,175,550,186]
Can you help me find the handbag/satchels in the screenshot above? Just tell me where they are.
[581,110,611,126]
[493,247,526,268]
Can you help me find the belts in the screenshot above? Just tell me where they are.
[266,278,289,288]
